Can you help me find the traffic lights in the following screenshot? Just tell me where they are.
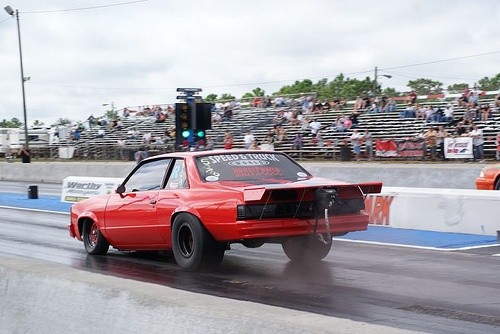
[176,102,211,146]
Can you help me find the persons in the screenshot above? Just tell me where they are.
[49,85,500,167]
[16,144,31,164]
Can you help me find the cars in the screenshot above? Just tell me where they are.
[67,149,383,272]
[476,161,500,190]
